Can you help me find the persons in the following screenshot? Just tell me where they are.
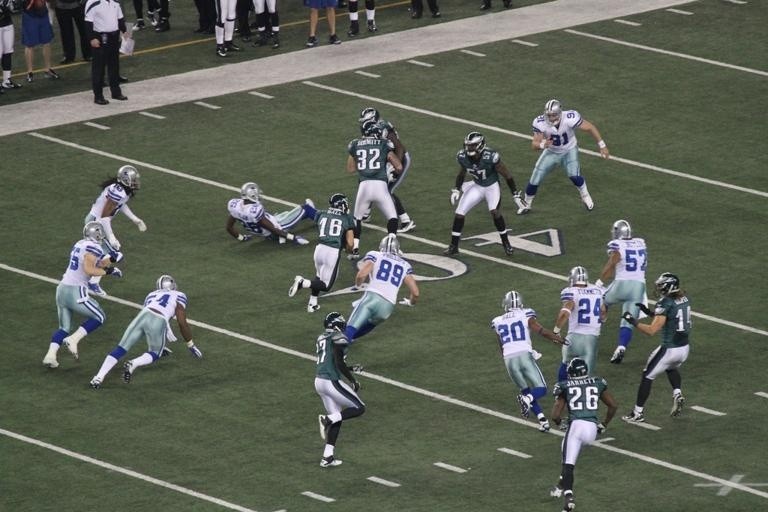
[517,98,610,215]
[443,132,530,257]
[348,0,379,37]
[621,272,693,423]
[49,0,93,64]
[155,0,171,33]
[252,0,281,48]
[84,0,131,105]
[491,289,570,432]
[594,219,649,364]
[85,0,128,87]
[214,0,241,57]
[287,192,357,313]
[411,0,441,18]
[0,0,23,90]
[551,356,618,512]
[18,0,61,83]
[552,266,607,380]
[89,274,203,390]
[358,108,417,233]
[42,221,123,369]
[346,120,404,261]
[314,312,367,467]
[303,0,342,46]
[479,0,513,10]
[132,0,162,31]
[225,181,318,245]
[345,235,421,340]
[84,165,148,297]
[236,0,256,42]
[193,0,217,34]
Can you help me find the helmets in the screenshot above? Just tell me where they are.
[567,358,588,377]
[364,122,383,138]
[156,274,176,291]
[464,133,487,163]
[544,100,561,125]
[656,272,681,296]
[84,221,107,242]
[570,267,588,285]
[503,290,521,312]
[379,234,401,256]
[240,182,263,203]
[330,193,348,216]
[359,108,377,125]
[611,220,631,240]
[325,312,344,332]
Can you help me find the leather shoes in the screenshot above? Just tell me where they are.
[114,95,126,101]
[120,74,129,82]
[480,4,491,10]
[413,11,421,18]
[96,96,107,104]
[436,11,442,16]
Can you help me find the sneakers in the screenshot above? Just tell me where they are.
[304,197,314,209]
[89,374,104,387]
[538,421,548,432]
[523,201,531,213]
[196,26,205,32]
[583,194,595,210]
[368,25,378,32]
[157,19,170,31]
[444,246,458,256]
[43,355,61,368]
[27,73,32,81]
[123,359,132,383]
[609,348,624,363]
[63,334,77,360]
[84,56,93,60]
[309,302,320,313]
[549,487,564,497]
[396,220,415,234]
[227,41,242,52]
[563,498,576,512]
[203,25,215,34]
[329,37,341,44]
[271,36,279,48]
[321,457,342,467]
[347,251,358,259]
[45,68,58,80]
[217,46,227,56]
[242,25,251,41]
[288,274,303,298]
[3,78,22,90]
[133,20,145,31]
[667,392,685,417]
[517,394,529,419]
[319,415,332,438]
[623,411,643,423]
[502,242,513,255]
[0,86,4,92]
[254,35,266,47]
[306,39,318,46]
[146,13,157,26]
[348,27,359,36]
[60,58,71,61]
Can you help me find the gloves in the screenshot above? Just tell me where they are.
[558,420,565,429]
[132,217,145,231]
[515,193,523,211]
[451,186,460,205]
[120,166,142,190]
[187,342,203,358]
[353,364,362,372]
[239,234,253,241]
[110,237,120,249]
[108,251,124,260]
[635,302,649,314]
[289,233,309,245]
[531,350,540,360]
[398,297,413,307]
[106,266,121,276]
[351,378,361,393]
[623,312,633,324]
[598,423,603,431]
